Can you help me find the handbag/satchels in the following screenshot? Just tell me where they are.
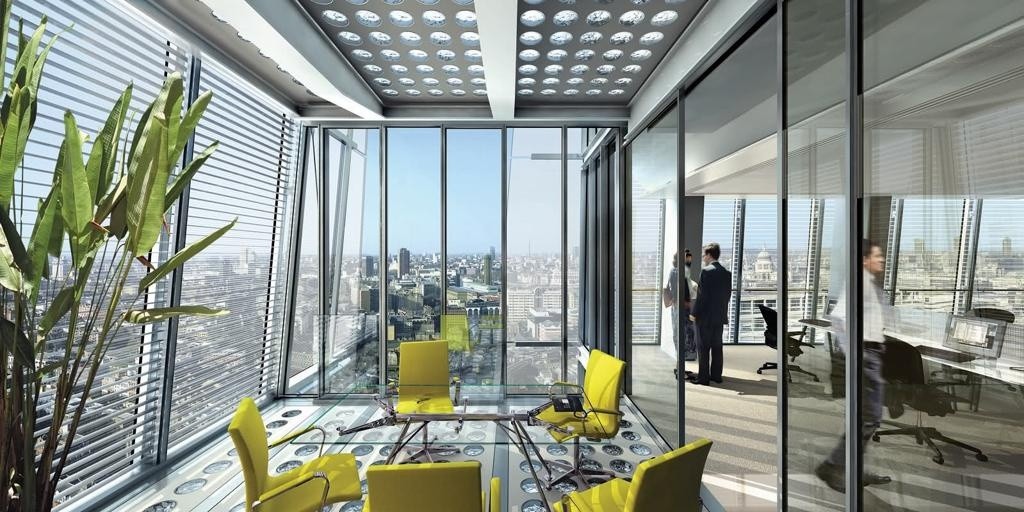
[664,288,672,307]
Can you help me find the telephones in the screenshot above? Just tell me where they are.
[553,395,583,412]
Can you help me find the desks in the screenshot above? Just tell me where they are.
[384,411,552,511]
[798,300,1024,411]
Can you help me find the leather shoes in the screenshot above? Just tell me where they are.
[674,369,723,386]
[815,463,891,493]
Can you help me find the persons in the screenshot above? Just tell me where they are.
[689,242,732,385]
[814,238,892,494]
[668,248,698,362]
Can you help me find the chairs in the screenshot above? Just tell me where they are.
[552,437,713,512]
[362,460,502,512]
[439,314,482,384]
[386,341,469,465]
[872,335,988,464]
[943,307,1015,413]
[228,398,362,511]
[478,315,504,350]
[757,304,819,382]
[527,349,626,492]
